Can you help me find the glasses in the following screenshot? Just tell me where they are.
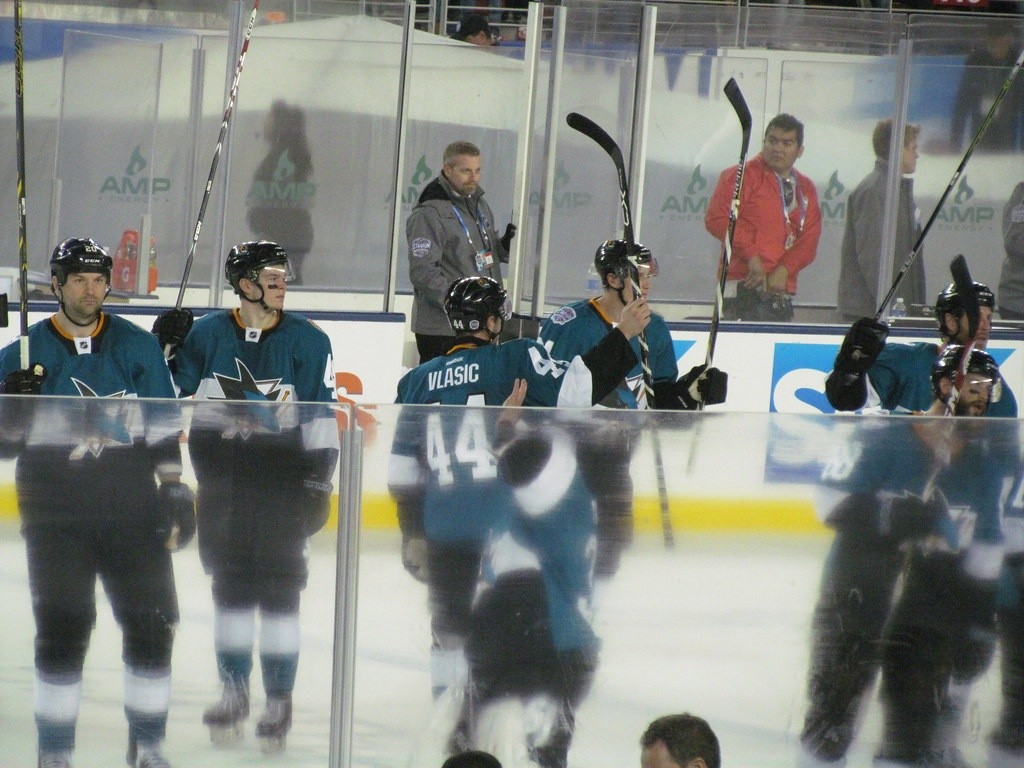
[783,178,794,206]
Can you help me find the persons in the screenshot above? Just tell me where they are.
[949,22,1024,153]
[801,281,1024,768]
[537,241,729,580]
[451,379,600,768]
[250,103,311,282]
[442,751,502,768]
[451,12,499,48]
[705,114,822,322]
[407,142,517,364]
[996,181,1024,320]
[641,714,721,768]
[152,241,338,754]
[838,118,926,321]
[1,238,196,768]
[387,275,651,699]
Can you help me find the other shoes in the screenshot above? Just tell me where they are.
[594,542,623,578]
[932,740,971,768]
[795,747,846,767]
[985,737,1024,768]
[874,756,934,768]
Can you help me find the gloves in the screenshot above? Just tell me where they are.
[154,481,195,549]
[952,620,997,682]
[502,224,516,251]
[284,470,333,539]
[825,317,889,411]
[151,308,194,361]
[401,532,429,582]
[676,363,728,408]
[0,362,47,430]
[877,496,947,540]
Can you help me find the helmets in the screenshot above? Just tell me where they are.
[929,345,1001,384]
[595,240,659,280]
[50,237,113,284]
[225,240,296,294]
[935,281,996,320]
[443,276,513,331]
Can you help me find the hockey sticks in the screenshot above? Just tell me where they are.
[851,49,1024,362]
[563,110,675,550]
[174,0,261,310]
[10,0,34,377]
[684,77,753,478]
[876,252,982,656]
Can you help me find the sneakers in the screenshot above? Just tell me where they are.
[255,695,292,752]
[202,682,250,743]
[125,738,170,768]
[38,748,73,768]
